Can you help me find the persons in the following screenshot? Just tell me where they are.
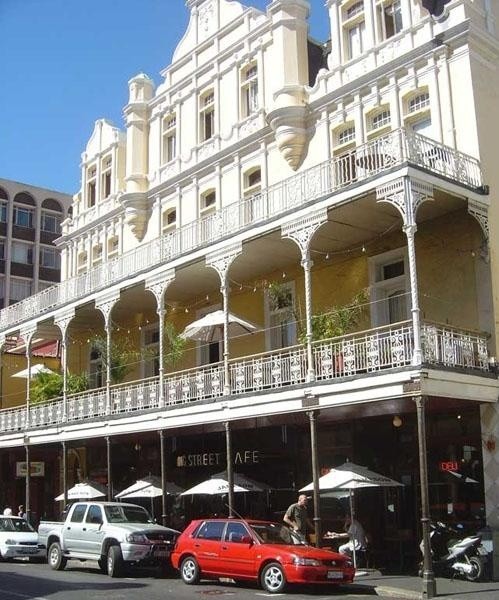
[17,504,27,520]
[3,504,13,515]
[338,512,367,560]
[282,495,316,543]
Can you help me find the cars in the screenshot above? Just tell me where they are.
[170,518,356,593]
[0,515,38,562]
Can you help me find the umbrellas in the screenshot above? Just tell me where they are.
[180,308,256,359]
[299,461,407,570]
[114,475,186,520]
[54,482,118,500]
[10,363,59,380]
[182,469,271,498]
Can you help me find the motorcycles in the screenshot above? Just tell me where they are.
[418,518,484,582]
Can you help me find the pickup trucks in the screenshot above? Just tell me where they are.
[37,501,183,577]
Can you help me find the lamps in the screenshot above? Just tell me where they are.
[393,414,402,427]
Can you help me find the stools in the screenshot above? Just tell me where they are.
[355,548,369,568]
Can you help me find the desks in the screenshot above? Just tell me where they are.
[323,536,349,551]
[383,536,412,569]
